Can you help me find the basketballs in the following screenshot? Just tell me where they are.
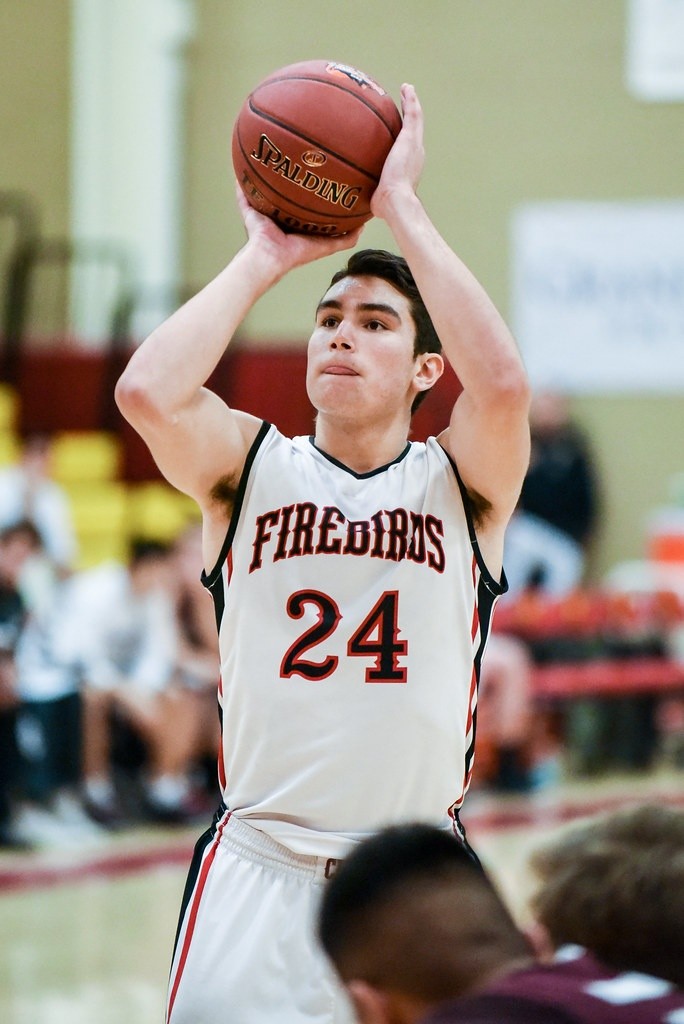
[231,57,403,238]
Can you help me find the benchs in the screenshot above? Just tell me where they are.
[533,658,682,704]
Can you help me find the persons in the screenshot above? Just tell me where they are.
[530,801,684,983]
[113,83,534,1023]
[0,385,597,852]
[322,823,684,1024]
